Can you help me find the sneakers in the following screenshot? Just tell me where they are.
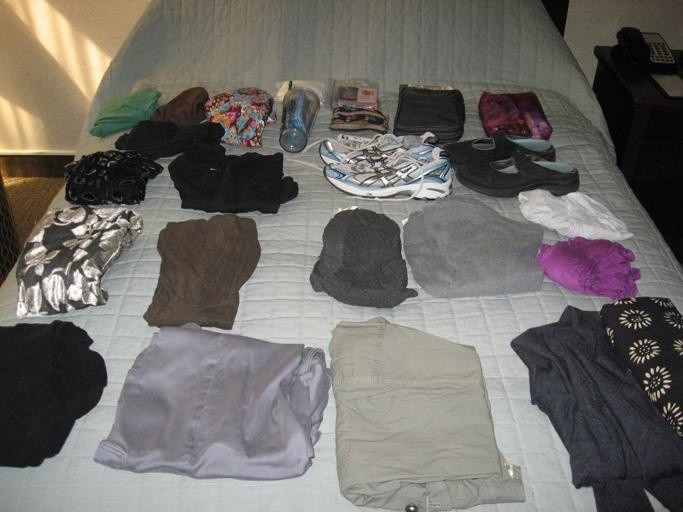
[318,105,454,202]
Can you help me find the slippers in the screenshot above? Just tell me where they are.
[445,133,580,198]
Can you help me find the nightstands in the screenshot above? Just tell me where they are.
[589,39,683,266]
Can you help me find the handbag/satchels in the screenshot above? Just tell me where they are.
[393,86,466,143]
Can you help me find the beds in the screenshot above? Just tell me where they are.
[0,1,683,512]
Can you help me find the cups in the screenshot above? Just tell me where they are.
[278,84,321,153]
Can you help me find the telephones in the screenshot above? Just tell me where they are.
[617,27,677,74]
